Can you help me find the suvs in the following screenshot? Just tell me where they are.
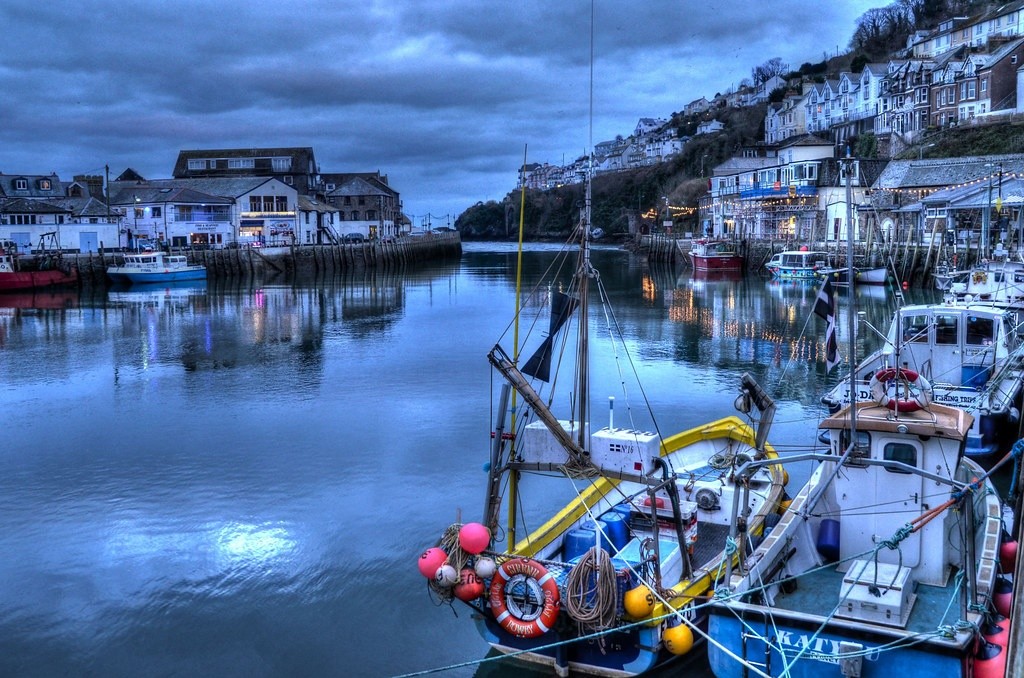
[341,233,364,243]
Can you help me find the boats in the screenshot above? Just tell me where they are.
[107,253,207,282]
[764,251,847,278]
[931,154,1024,313]
[707,144,1017,678]
[0,239,81,288]
[688,233,744,271]
[820,288,1024,457]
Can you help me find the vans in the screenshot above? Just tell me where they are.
[128,238,152,251]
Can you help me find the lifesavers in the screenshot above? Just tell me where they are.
[489,559,560,635]
[870,368,933,412]
[973,271,986,284]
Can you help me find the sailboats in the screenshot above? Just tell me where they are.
[419,0,793,678]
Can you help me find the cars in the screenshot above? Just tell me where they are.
[382,234,395,243]
[409,231,425,236]
